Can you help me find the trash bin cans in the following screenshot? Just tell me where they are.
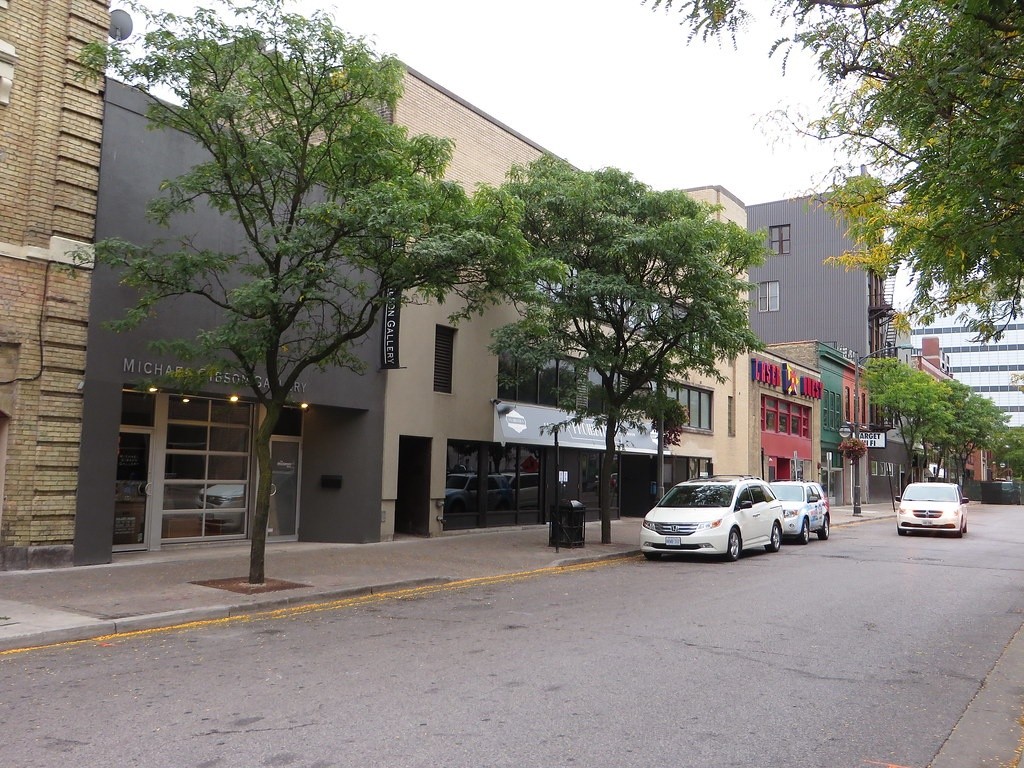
[548,498,585,548]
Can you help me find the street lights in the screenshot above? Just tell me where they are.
[839,344,913,514]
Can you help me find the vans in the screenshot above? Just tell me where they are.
[639,474,782,561]
[894,481,970,537]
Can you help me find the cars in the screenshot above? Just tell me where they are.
[445,473,515,513]
[273,461,295,476]
[500,473,540,510]
[196,484,247,533]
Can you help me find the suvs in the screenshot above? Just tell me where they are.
[769,478,831,544]
